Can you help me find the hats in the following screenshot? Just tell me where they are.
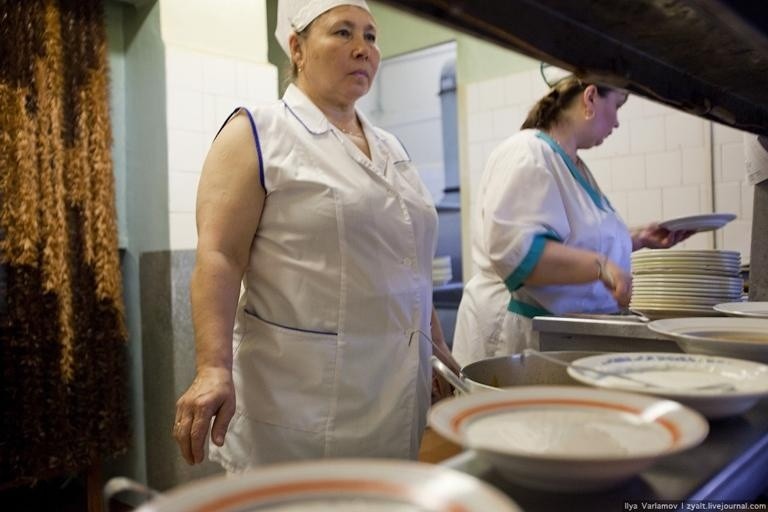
[274,0,376,60]
[541,61,573,87]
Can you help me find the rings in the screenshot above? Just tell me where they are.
[176,422,180,426]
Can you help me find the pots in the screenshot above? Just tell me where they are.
[431,348,604,397]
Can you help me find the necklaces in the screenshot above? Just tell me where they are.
[552,139,582,169]
[330,122,365,139]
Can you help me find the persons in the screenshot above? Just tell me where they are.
[171,2,465,475]
[451,60,695,375]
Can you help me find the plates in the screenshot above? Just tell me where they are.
[628,248,743,321]
[426,385,711,495]
[431,255,453,285]
[660,213,737,233]
[713,301,768,318]
[647,316,768,360]
[133,457,525,512]
[566,352,768,420]
